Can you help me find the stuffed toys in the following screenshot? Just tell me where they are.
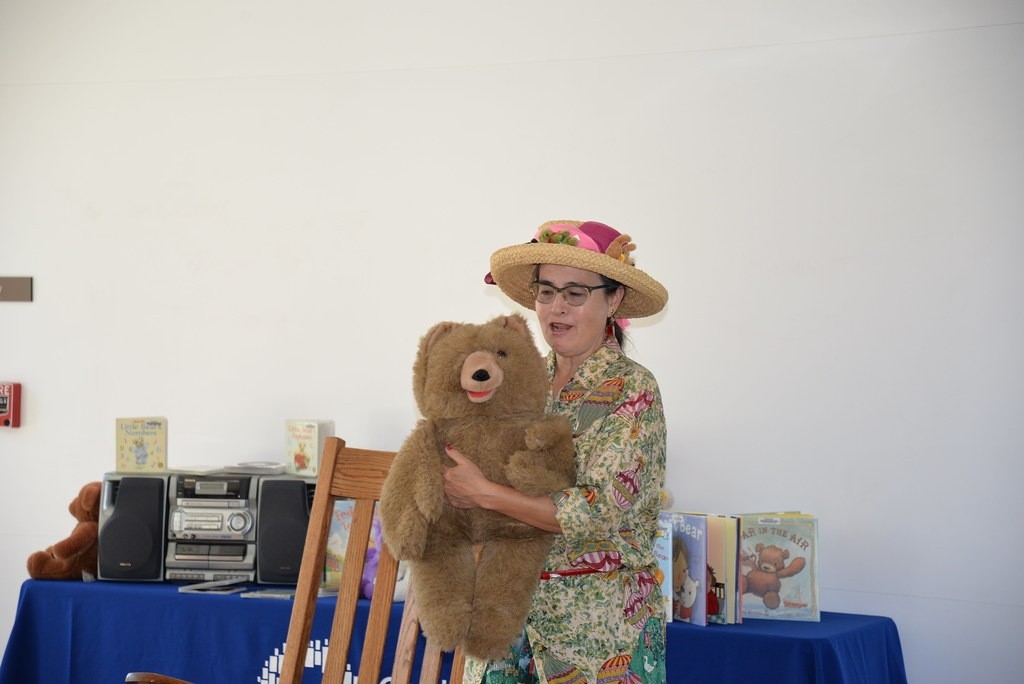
[24,481,102,583]
[376,312,581,664]
[361,520,410,602]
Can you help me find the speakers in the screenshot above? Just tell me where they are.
[258,474,327,589]
[97,470,170,582]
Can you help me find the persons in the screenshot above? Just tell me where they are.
[459,217,673,683]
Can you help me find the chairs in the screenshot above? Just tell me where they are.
[126,438,468,684]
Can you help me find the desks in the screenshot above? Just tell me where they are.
[0,580,908,684]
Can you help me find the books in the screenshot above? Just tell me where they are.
[285,417,337,479]
[654,504,824,625]
[115,416,169,473]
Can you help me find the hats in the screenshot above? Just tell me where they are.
[485,220,669,318]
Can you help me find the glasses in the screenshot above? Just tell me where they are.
[528,269,609,306]
